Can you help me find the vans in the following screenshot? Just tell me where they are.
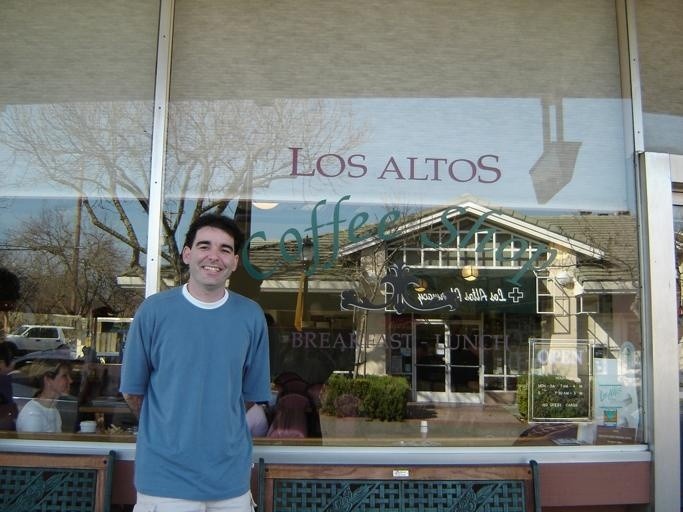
[1,325,75,361]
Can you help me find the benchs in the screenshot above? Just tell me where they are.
[0,450,116,512]
[258,458,541,512]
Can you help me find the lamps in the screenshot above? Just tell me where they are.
[414,278,427,292]
[557,268,573,285]
[461,265,479,281]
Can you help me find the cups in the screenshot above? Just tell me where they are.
[79,419,97,433]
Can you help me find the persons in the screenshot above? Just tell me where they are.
[0,339,307,440]
[119,214,272,512]
